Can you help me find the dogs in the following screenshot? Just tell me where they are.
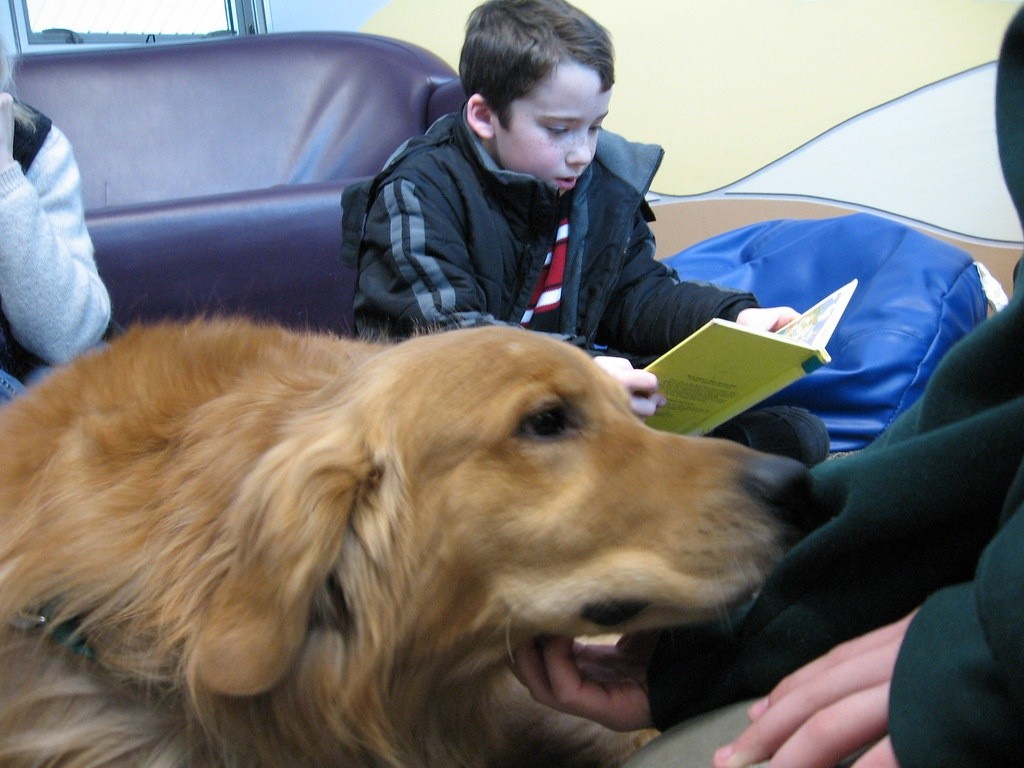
[0,314,816,768]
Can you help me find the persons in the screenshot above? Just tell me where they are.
[0,40,113,417]
[506,0,1024,768]
[342,0,805,428]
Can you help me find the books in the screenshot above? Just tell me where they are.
[642,275,859,438]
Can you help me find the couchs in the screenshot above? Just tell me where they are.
[0,32,1024,470]
